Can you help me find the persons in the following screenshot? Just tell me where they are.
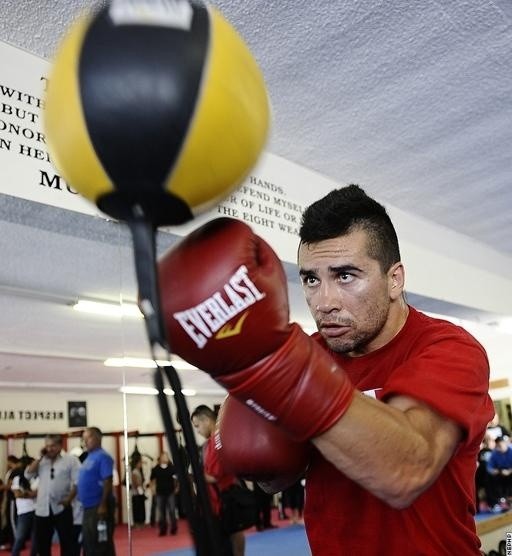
[475,413,511,516]
[1,406,307,555]
[159,182,496,556]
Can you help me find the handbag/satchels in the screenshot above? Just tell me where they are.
[218,484,256,534]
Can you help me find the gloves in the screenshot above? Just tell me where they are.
[203,394,310,496]
[137,218,353,441]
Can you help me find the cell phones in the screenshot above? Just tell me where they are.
[41,446,48,456]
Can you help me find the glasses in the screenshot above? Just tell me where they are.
[51,468,54,478]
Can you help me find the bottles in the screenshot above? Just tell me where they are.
[97,518,108,541]
